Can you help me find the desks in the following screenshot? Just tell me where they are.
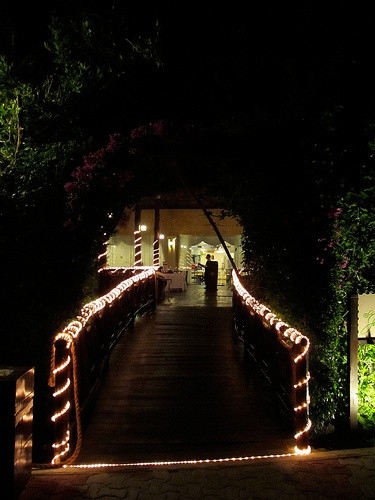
[162,273,185,291]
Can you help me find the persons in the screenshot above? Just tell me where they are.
[203,254,211,289]
